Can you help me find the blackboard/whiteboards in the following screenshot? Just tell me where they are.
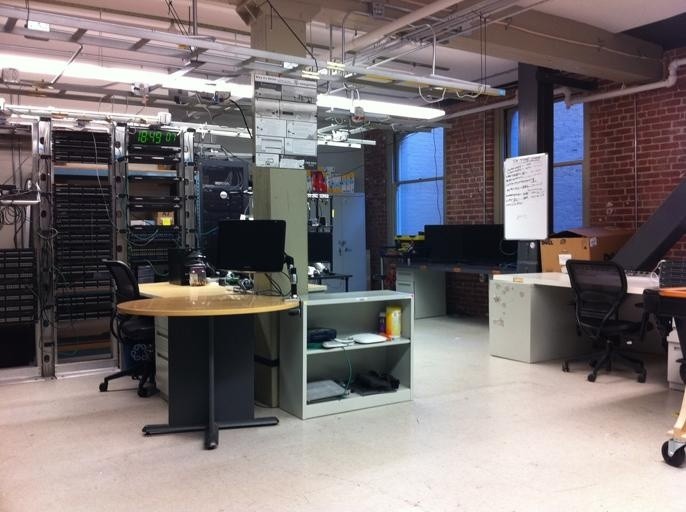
[502,152,549,241]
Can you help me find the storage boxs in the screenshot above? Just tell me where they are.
[540,226,626,273]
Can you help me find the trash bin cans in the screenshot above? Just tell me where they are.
[121,337,155,371]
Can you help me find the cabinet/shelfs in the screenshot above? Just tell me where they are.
[278,289,414,420]
[309,192,367,293]
[396,268,446,319]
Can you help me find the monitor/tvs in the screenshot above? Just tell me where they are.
[424,224,518,268]
[214,219,286,272]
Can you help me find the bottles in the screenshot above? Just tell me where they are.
[188,264,208,285]
[378,313,386,335]
[311,170,327,193]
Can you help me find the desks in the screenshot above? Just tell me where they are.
[117,281,299,450]
[489,272,685,392]
[308,272,352,291]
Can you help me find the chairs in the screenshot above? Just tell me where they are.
[100,258,155,398]
[562,259,654,383]
